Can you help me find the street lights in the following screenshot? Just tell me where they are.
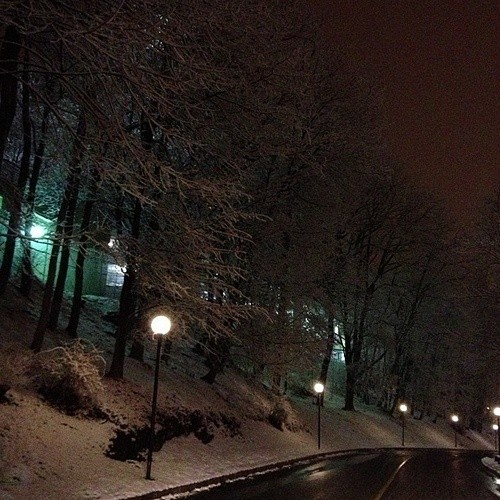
[493,407,500,450]
[314,383,324,449]
[452,416,458,447]
[493,424,498,450]
[144,315,171,480]
[399,405,407,447]
